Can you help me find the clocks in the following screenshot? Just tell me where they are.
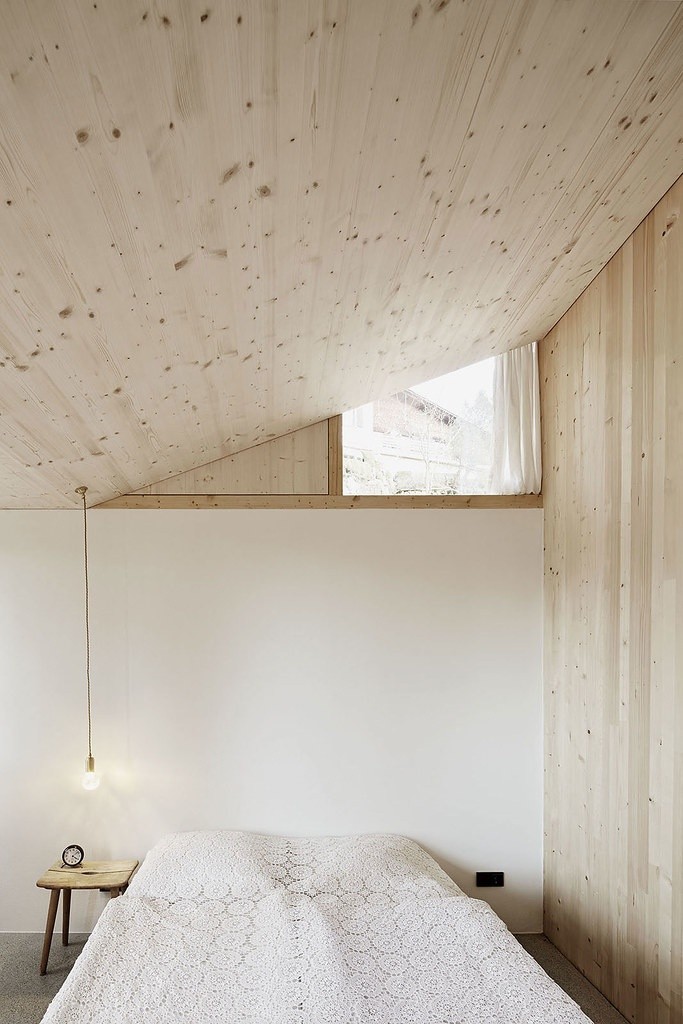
[61,845,84,869]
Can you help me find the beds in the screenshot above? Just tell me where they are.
[36,831,596,1024]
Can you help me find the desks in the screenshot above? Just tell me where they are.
[37,860,139,977]
